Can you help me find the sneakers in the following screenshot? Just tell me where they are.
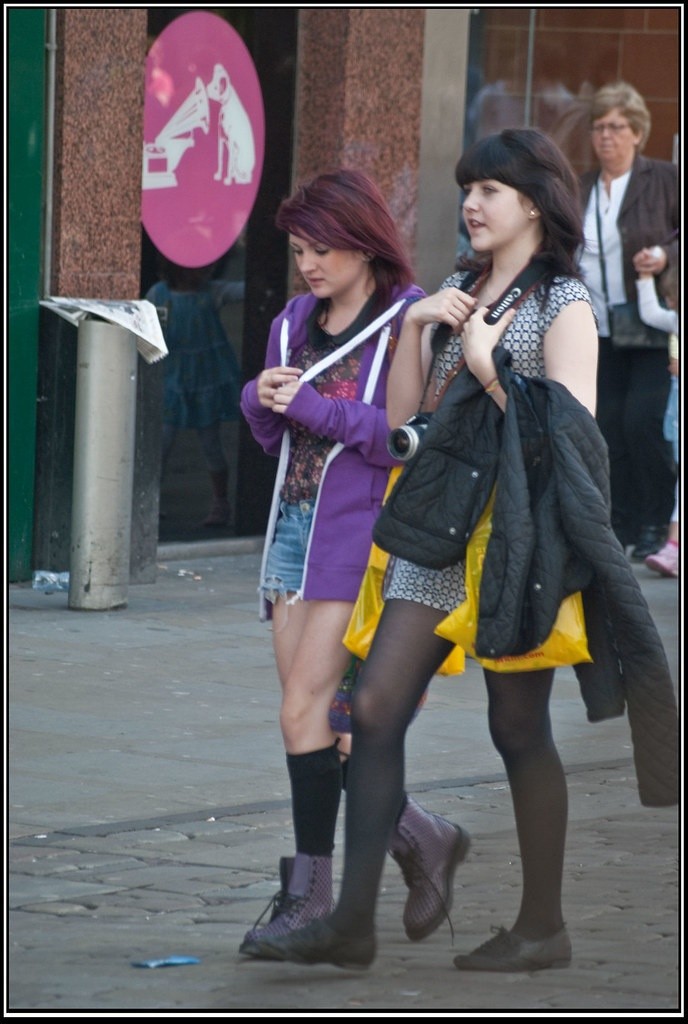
[644,540,679,576]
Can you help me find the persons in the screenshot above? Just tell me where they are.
[240,168,472,960]
[575,82,679,579]
[237,127,599,971]
[146,261,245,533]
[464,45,574,139]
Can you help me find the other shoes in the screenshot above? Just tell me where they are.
[630,522,669,560]
[241,916,377,971]
[453,922,572,973]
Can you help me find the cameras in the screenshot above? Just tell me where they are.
[388,412,436,465]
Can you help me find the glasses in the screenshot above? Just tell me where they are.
[593,121,635,136]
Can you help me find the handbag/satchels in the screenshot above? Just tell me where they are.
[608,300,671,348]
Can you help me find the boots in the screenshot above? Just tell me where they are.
[239,854,333,960]
[388,790,473,941]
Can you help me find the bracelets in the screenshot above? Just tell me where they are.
[484,378,500,396]
